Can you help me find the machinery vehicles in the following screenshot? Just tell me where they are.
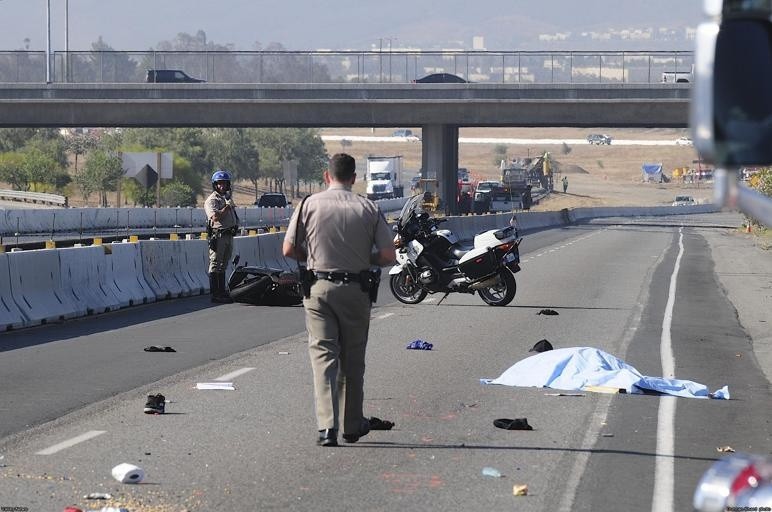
[500,152,554,189]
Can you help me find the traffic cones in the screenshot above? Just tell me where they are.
[745,220,752,233]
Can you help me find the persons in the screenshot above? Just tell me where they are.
[561,176,569,193]
[280,152,397,449]
[203,170,239,304]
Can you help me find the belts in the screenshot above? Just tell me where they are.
[313,271,362,282]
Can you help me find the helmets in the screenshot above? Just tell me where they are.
[211,170,231,184]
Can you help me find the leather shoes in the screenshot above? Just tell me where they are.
[341,416,372,443]
[316,429,338,446]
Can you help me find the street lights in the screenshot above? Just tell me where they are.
[377,37,384,81]
[386,37,398,80]
[23,36,31,55]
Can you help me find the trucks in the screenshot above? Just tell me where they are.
[365,153,405,200]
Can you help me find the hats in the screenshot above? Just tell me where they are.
[533,339,554,353]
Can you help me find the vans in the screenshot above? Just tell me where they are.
[391,128,412,137]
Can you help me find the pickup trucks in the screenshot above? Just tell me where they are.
[661,63,695,84]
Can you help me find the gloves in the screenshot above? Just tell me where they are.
[225,198,235,207]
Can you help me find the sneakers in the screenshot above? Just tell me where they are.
[143,393,167,415]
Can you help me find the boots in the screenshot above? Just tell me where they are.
[207,270,234,304]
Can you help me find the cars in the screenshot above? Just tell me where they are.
[411,168,551,216]
[682,166,761,184]
[675,135,694,147]
[405,134,423,142]
[412,74,472,82]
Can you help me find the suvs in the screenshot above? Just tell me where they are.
[144,69,204,82]
[673,194,695,207]
[586,134,612,146]
[255,192,293,214]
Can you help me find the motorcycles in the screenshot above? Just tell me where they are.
[226,253,303,305]
[388,193,523,308]
